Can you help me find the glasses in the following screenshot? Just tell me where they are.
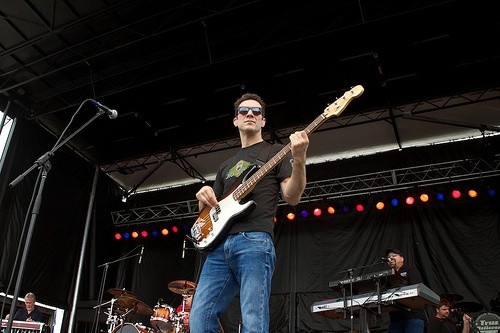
[238,106,262,116]
[386,255,398,258]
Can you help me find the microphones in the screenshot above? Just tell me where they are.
[381,257,391,262]
[139,247,144,263]
[182,240,186,258]
[451,308,461,312]
[89,99,118,119]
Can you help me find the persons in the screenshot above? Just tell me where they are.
[177,293,194,315]
[427,299,472,333]
[378,248,426,333]
[5,293,49,324]
[189,94,310,333]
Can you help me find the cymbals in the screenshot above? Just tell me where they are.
[167,279,196,296]
[116,296,150,316]
[105,289,136,298]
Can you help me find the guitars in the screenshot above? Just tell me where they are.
[185,83,364,254]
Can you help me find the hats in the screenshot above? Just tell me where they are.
[385,247,402,255]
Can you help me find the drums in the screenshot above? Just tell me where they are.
[111,323,156,333]
[170,312,190,333]
[147,303,176,330]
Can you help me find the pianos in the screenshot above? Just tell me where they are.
[310,268,442,333]
